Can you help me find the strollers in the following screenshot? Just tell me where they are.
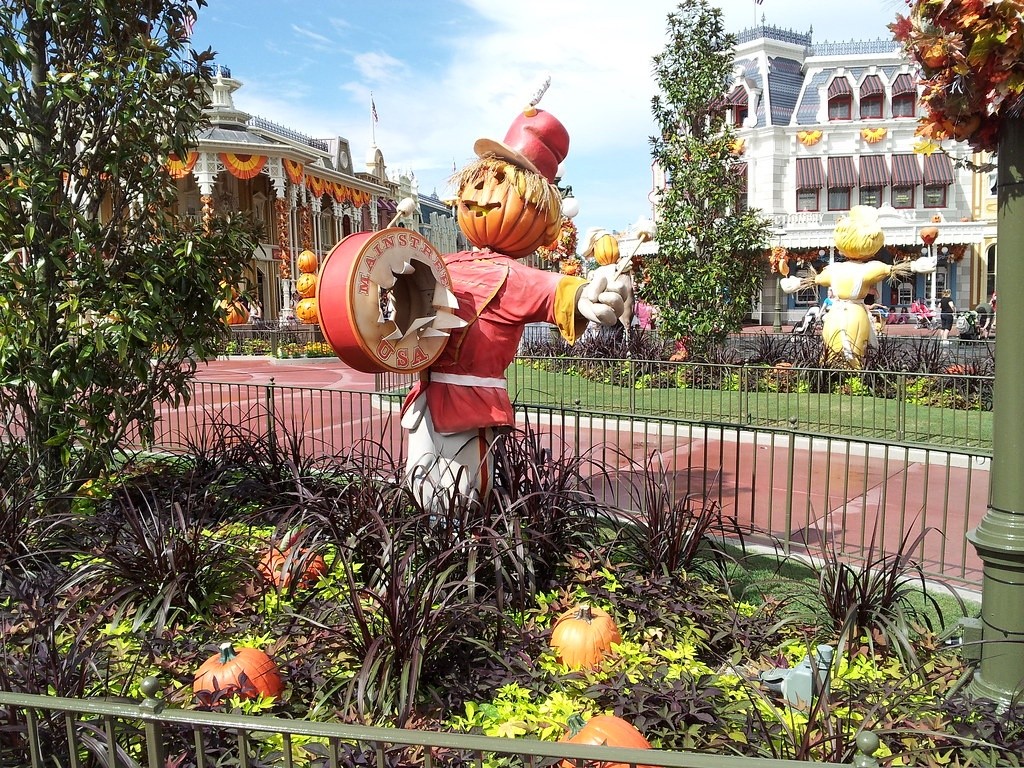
[790,305,822,342]
[956,311,983,346]
[870,305,889,345]
[913,298,942,331]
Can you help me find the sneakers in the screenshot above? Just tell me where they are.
[940,340,953,345]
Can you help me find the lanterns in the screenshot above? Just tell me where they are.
[295,250,320,324]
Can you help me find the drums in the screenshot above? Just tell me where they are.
[315,227,469,374]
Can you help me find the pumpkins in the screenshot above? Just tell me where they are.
[553,714,664,768]
[551,603,619,672]
[193,641,283,702]
[246,524,326,596]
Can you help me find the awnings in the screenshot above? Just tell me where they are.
[705,85,748,112]
[796,153,956,191]
[377,197,397,213]
[828,74,918,101]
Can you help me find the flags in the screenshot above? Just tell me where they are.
[372,99,378,123]
[182,10,196,40]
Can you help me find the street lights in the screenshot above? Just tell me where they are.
[772,228,787,333]
[921,247,948,321]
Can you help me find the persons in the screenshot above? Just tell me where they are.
[231,282,262,324]
[888,289,997,339]
[289,290,304,319]
[781,206,935,358]
[818,286,887,322]
